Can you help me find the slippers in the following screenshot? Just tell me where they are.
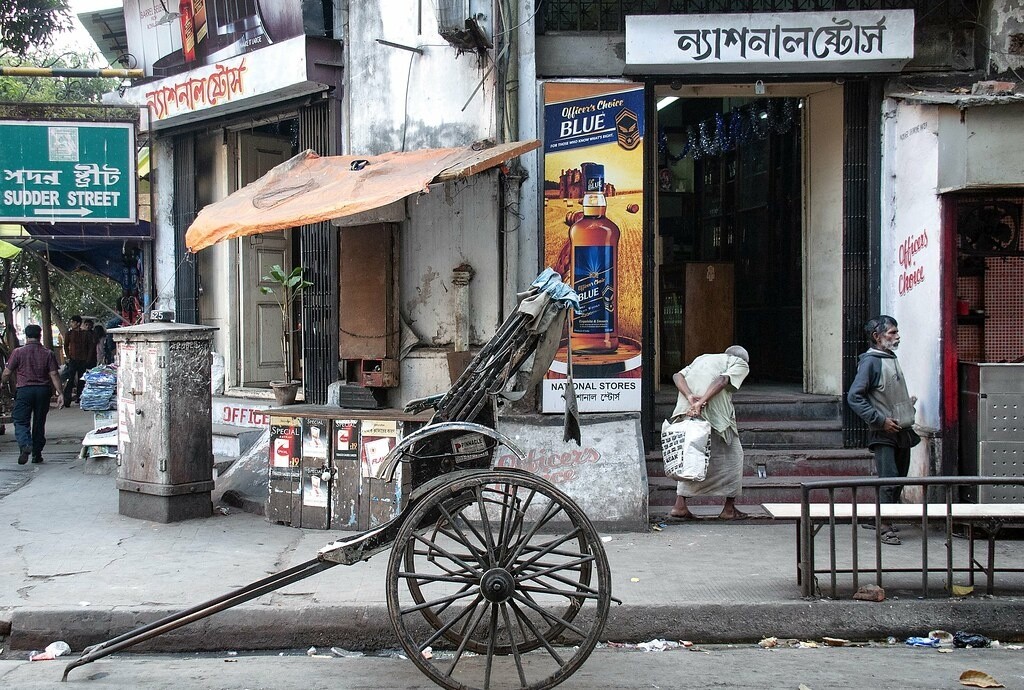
[668,510,705,521]
[718,505,747,520]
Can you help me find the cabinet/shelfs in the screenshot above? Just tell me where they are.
[661,260,736,382]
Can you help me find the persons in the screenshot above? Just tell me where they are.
[60,315,90,407]
[105,296,124,365]
[2,325,66,466]
[82,318,98,372]
[847,315,920,547]
[667,345,751,523]
[93,326,108,365]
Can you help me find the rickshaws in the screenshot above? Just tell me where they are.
[61,268,622,690]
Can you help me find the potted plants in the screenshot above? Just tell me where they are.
[256,262,315,406]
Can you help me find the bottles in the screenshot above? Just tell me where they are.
[569,164,619,355]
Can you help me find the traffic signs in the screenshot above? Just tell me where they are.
[0,118,138,225]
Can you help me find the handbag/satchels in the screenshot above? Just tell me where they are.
[661,409,711,482]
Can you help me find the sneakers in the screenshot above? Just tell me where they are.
[31,455,44,463]
[18,444,32,464]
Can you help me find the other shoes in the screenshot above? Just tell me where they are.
[76,398,80,402]
[64,401,72,406]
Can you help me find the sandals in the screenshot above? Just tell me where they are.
[881,526,901,544]
[873,524,899,532]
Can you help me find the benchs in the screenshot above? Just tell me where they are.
[761,500,1024,597]
